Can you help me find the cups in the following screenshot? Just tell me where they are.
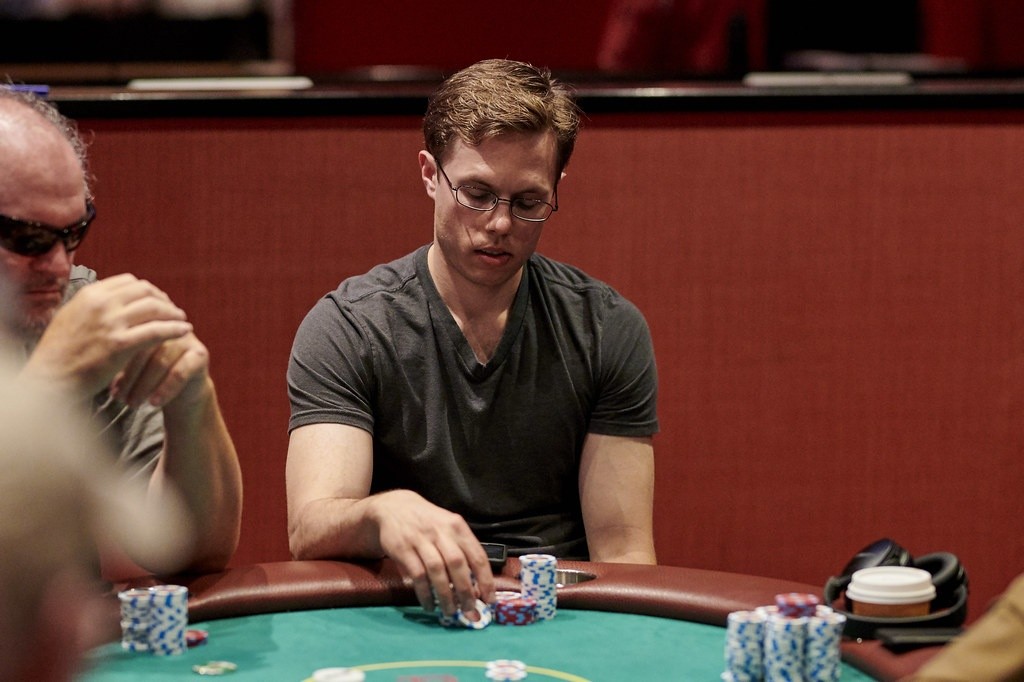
[845,566,937,617]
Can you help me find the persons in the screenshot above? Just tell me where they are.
[285,56,659,616]
[0,86,243,682]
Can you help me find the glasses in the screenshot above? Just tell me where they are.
[0,197,97,258]
[436,158,558,222]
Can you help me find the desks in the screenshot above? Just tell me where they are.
[73,558,948,682]
[20,74,1024,624]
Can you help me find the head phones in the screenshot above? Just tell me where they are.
[825,536,970,640]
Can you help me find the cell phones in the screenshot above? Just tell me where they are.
[478,542,507,567]
